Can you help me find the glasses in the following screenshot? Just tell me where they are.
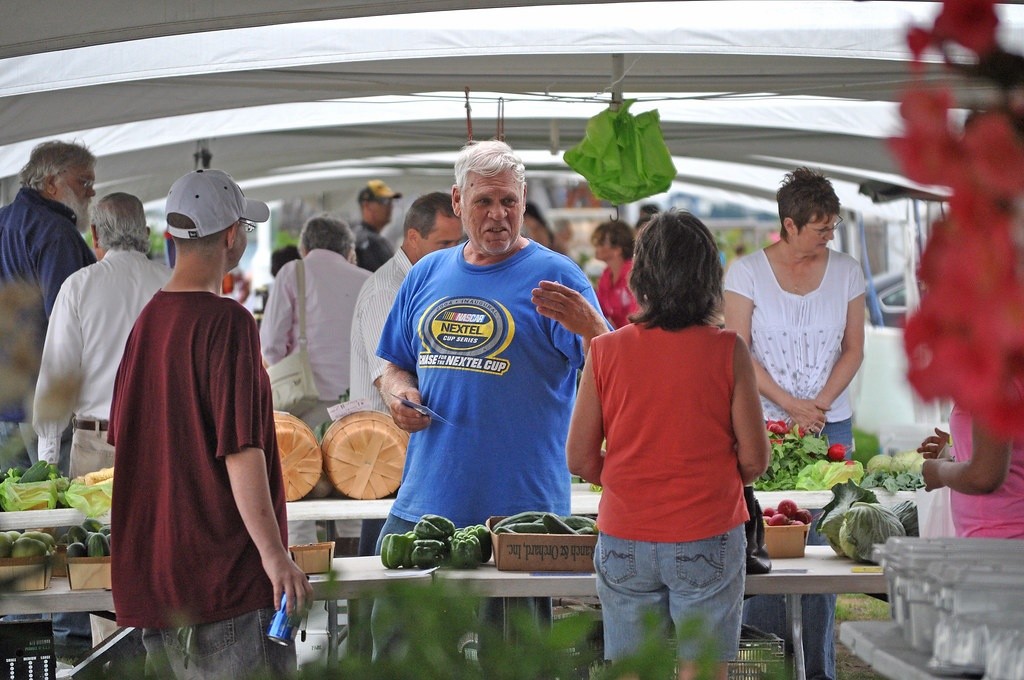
[237,219,256,232]
[801,215,843,235]
[372,197,392,205]
[57,171,94,190]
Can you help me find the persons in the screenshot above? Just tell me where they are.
[0,140,97,667]
[33,193,174,650]
[916,400,1024,540]
[589,220,642,328]
[373,142,614,661]
[566,210,771,680]
[723,167,865,680]
[522,206,573,254]
[350,191,463,556]
[106,169,314,680]
[353,179,403,272]
[259,214,375,546]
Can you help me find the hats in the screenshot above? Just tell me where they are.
[358,179,402,205]
[165,168,271,238]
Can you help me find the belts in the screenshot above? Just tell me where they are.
[74,419,108,431]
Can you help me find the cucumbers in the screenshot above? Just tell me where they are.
[65,518,114,557]
[493,511,598,536]
[0,460,61,484]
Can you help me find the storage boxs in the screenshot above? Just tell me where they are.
[0,554,54,592]
[870,537,1024,680]
[762,515,811,558]
[64,556,112,590]
[585,619,787,680]
[485,516,598,571]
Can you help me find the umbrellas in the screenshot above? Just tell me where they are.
[0,0,1024,224]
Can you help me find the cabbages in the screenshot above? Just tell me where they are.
[816,477,920,564]
[867,450,927,475]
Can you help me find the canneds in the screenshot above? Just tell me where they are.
[266,589,302,646]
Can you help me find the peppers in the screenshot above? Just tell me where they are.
[380,514,491,570]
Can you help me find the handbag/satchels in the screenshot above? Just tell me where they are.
[264,346,319,418]
[742,484,771,574]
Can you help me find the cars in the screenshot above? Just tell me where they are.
[856,270,923,329]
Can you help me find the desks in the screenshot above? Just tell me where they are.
[0,488,994,680]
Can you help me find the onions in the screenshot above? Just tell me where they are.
[761,498,812,528]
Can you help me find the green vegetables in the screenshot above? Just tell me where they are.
[860,473,927,493]
